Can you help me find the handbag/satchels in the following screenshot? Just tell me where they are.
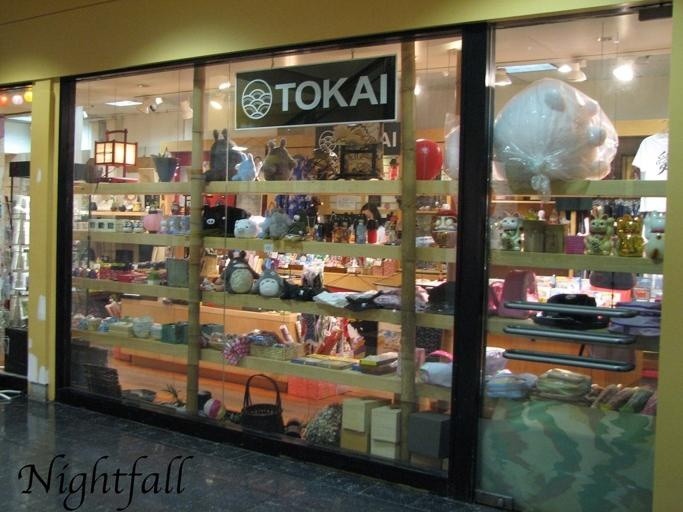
[418,351,453,388]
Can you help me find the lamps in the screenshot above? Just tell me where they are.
[94,131,139,166]
[565,62,586,82]
[208,92,226,110]
[180,100,194,120]
[494,66,512,86]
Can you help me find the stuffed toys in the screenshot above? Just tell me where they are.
[202,205,245,236]
[499,212,522,253]
[583,216,614,256]
[232,153,261,182]
[234,215,265,239]
[218,252,253,293]
[302,141,383,183]
[643,210,665,260]
[614,214,644,259]
[261,139,299,181]
[203,129,242,183]
[255,259,283,296]
[493,77,608,183]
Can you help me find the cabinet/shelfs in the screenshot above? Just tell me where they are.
[70,181,681,512]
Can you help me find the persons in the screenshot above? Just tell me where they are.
[357,203,383,246]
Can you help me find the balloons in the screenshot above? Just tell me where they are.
[414,139,441,181]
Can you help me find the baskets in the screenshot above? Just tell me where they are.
[606,386,639,410]
[619,388,653,413]
[590,384,618,408]
[640,391,658,414]
[241,374,283,433]
